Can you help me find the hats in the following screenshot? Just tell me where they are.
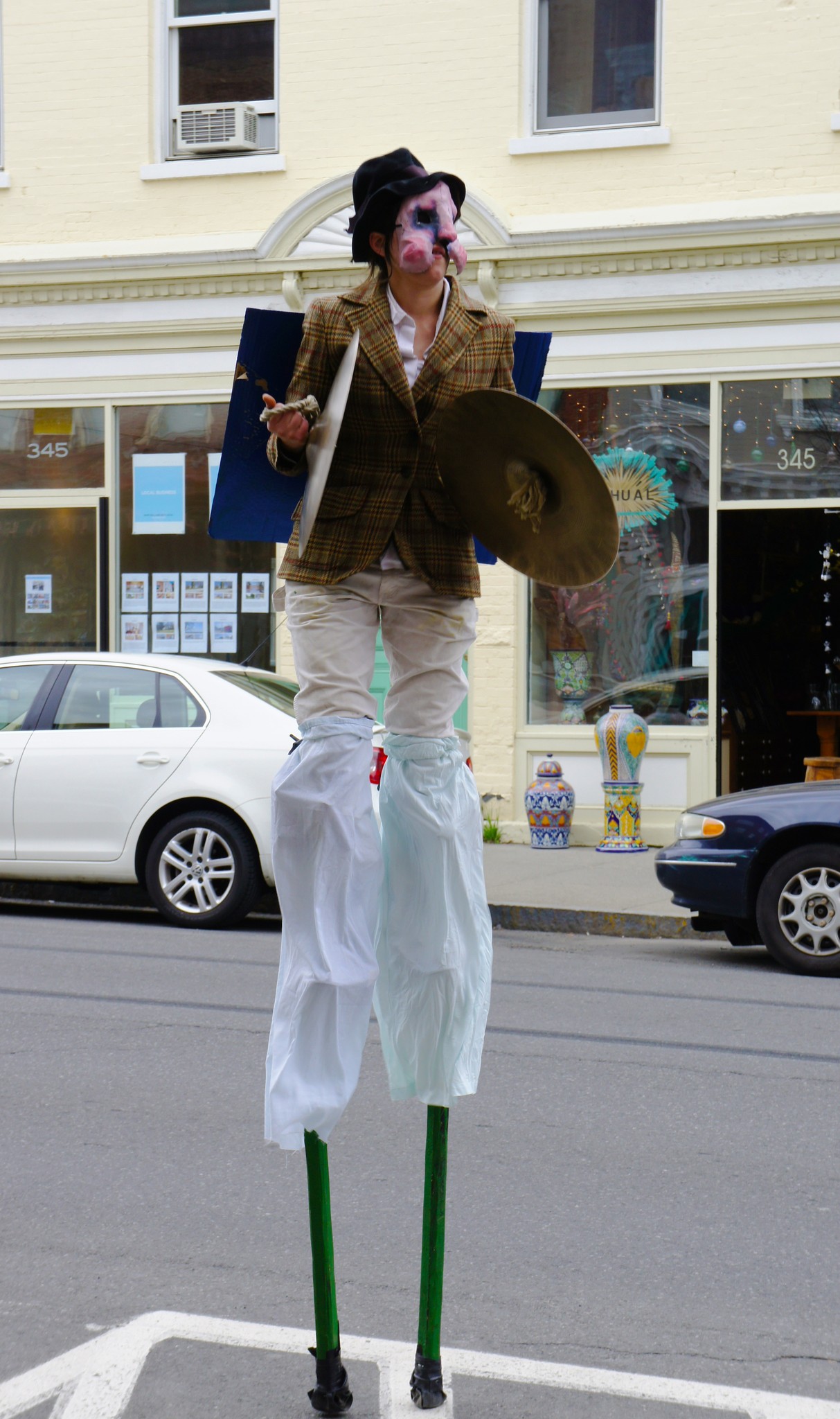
[351,150,466,264]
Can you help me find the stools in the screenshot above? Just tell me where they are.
[803,751,840,783]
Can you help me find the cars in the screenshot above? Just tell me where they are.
[1,652,475,929]
[651,779,840,980]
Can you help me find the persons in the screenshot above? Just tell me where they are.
[262,152,515,1152]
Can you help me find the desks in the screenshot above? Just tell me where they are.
[785,709,840,757]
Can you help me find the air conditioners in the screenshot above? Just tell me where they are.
[175,101,260,154]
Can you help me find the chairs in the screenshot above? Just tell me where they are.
[67,686,102,727]
[133,694,161,728]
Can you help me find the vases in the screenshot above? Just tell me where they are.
[591,701,653,856]
[525,752,577,849]
[546,647,597,726]
